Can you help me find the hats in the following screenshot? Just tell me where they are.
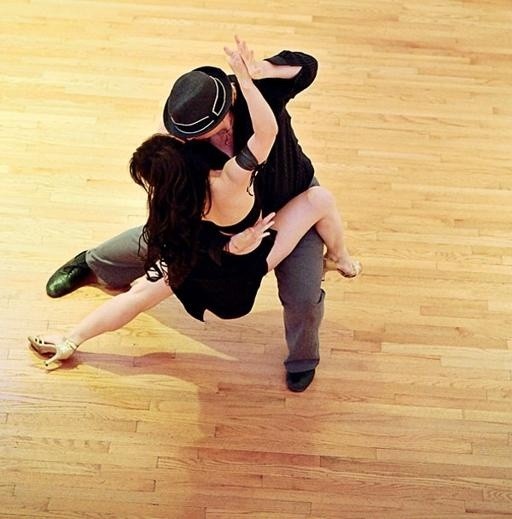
[163,66,231,139]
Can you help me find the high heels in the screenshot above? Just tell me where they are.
[28,333,77,366]
[322,256,363,281]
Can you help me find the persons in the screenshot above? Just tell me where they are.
[46,33,327,393]
[28,45,363,366]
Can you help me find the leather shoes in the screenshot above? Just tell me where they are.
[286,368,315,392]
[47,253,88,298]
